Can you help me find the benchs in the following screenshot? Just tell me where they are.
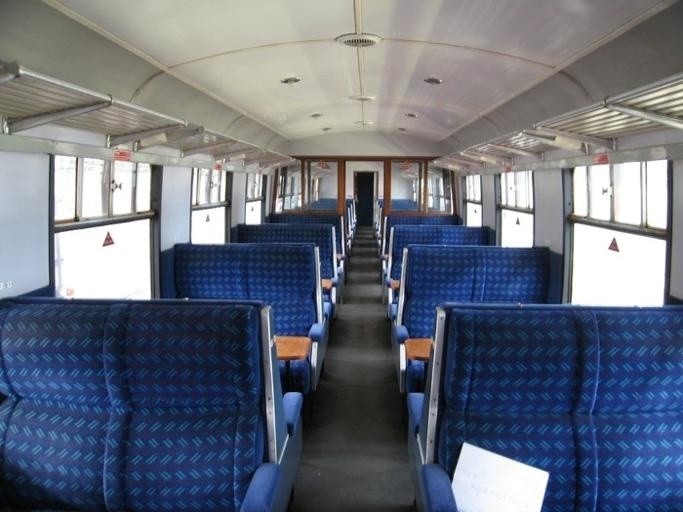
[374,197,681,510]
[1,197,357,511]
